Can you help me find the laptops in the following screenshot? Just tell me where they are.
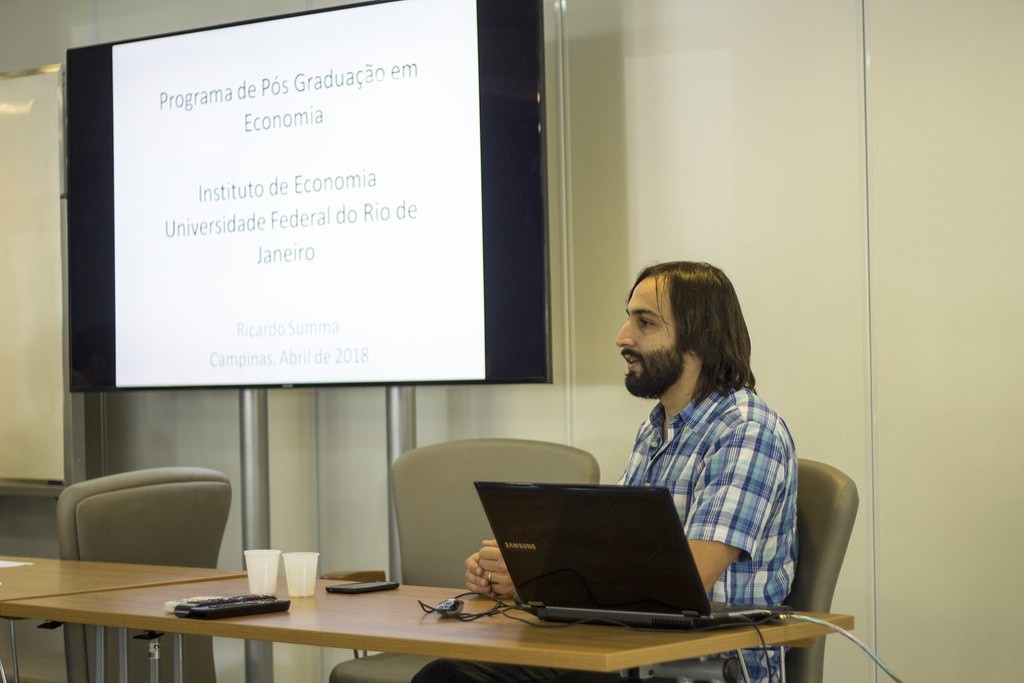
[473,481,794,631]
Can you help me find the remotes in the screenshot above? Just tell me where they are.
[165,595,291,620]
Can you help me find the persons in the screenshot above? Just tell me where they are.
[408,262,798,683]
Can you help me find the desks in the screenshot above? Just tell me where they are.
[4,578,854,683]
[0,554,246,683]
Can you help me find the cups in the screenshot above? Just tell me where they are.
[282,552,319,597]
[244,550,281,596]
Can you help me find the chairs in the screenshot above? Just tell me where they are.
[326,438,598,683]
[57,467,233,683]
[786,457,861,683]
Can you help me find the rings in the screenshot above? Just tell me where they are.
[489,571,491,581]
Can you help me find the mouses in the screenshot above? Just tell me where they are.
[436,598,465,616]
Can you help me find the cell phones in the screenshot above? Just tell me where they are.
[325,581,400,594]
